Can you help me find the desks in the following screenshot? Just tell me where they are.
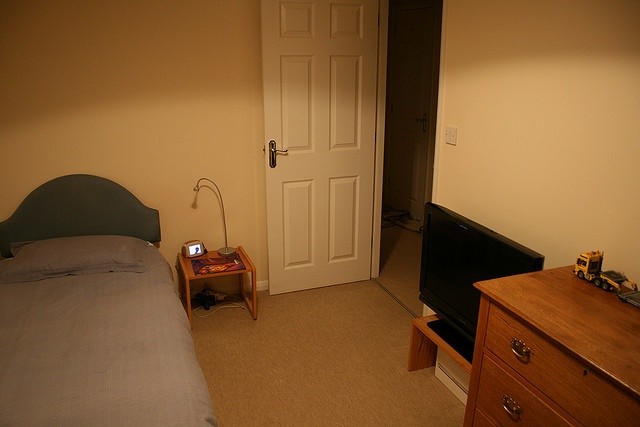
[406,313,472,376]
[174,244,258,330]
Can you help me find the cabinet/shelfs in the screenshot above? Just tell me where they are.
[462,272,640,425]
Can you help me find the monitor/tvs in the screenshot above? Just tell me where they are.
[419,201,545,364]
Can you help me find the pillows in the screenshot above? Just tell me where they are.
[0,233,148,283]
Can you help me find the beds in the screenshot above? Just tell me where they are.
[1,174,215,425]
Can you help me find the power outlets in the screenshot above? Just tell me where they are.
[444,129,456,144]
